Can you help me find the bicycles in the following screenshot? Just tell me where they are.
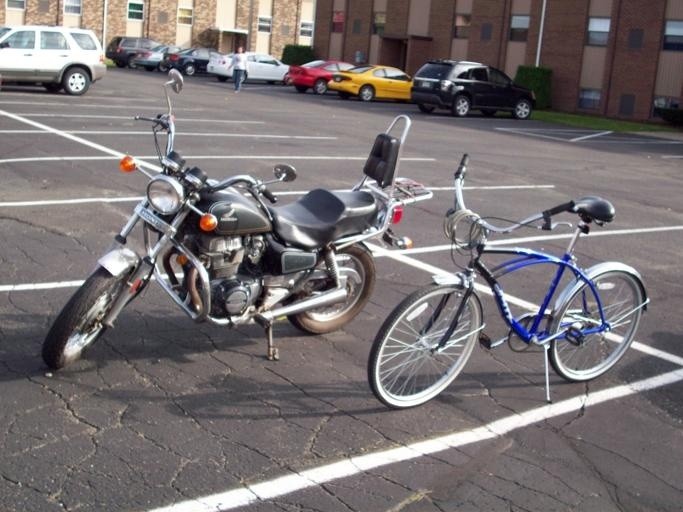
[367,152,648,408]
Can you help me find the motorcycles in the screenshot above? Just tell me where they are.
[40,68,435,371]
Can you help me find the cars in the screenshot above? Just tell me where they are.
[326,63,414,101]
[166,48,225,75]
[205,51,291,83]
[283,59,358,94]
[134,44,180,72]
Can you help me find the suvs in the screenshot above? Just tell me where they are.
[411,59,536,121]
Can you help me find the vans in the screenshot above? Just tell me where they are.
[106,35,161,68]
[0,25,106,94]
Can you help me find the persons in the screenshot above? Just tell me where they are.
[226,42,250,94]
